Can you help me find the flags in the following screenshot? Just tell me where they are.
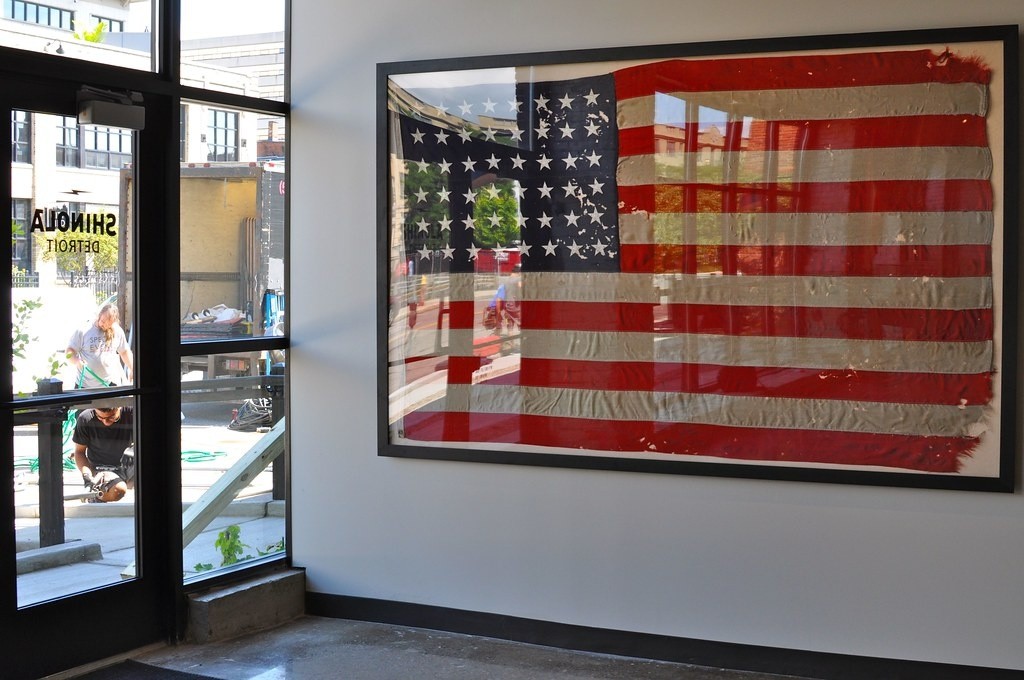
[349,24,1024,497]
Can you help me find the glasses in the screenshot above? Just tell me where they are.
[95,412,117,420]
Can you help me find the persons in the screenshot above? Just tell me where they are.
[502,265,521,357]
[66,304,136,392]
[485,283,506,337]
[73,407,137,504]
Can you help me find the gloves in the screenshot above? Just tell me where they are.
[83,473,96,488]
